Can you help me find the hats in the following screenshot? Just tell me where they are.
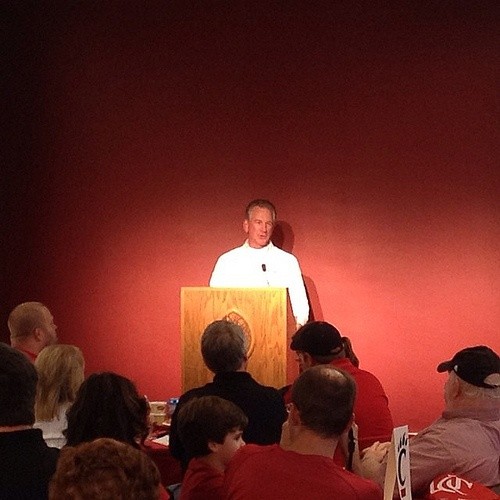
[437,345,500,389]
[290,321,343,356]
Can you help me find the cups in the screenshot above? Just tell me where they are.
[150,402,167,414]
[150,414,166,424]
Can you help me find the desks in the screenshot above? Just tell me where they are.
[135,424,183,484]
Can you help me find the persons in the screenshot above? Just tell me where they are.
[7,302,56,358]
[209,200,310,334]
[384,346,500,499]
[295,337,359,371]
[170,321,287,446]
[32,345,84,429]
[225,366,384,500]
[169,398,246,499]
[60,372,150,446]
[281,322,394,457]
[0,345,59,500]
[49,439,162,500]
